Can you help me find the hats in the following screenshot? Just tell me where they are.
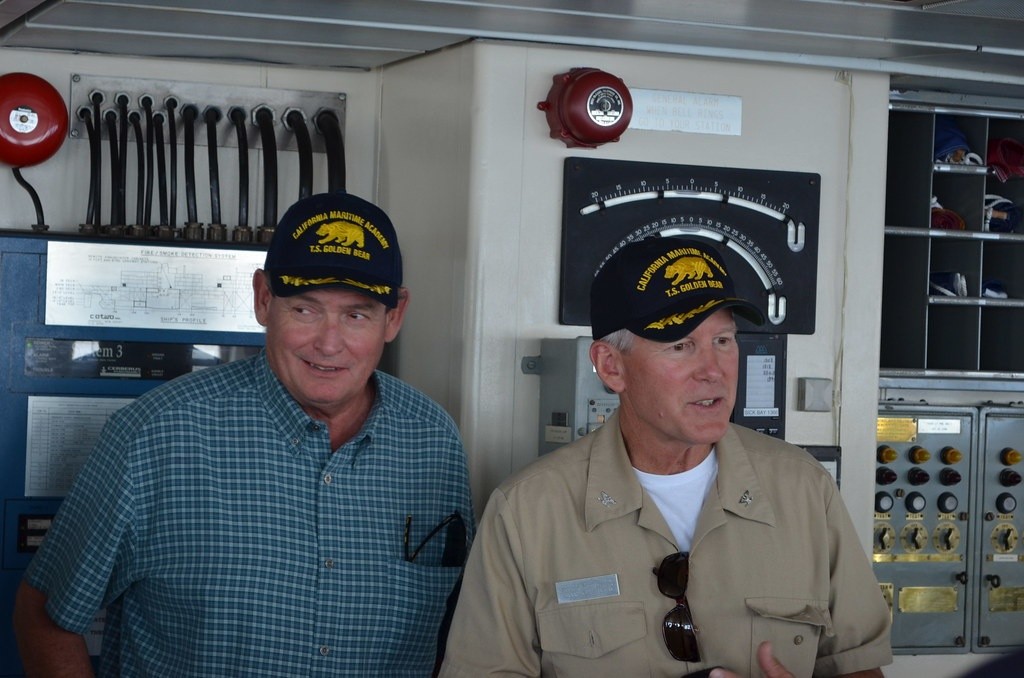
[589,238,765,343]
[263,192,403,308]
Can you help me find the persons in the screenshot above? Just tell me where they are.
[10,190,477,678]
[438,237,895,678]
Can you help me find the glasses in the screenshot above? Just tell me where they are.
[405,511,466,568]
[652,551,702,662]
